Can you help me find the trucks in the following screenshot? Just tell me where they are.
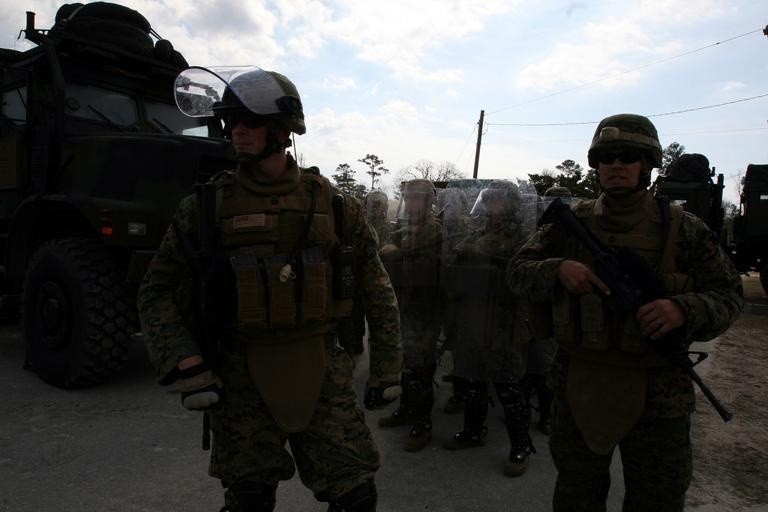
[656,165,768,295]
[0,40,238,391]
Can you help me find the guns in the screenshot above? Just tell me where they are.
[174,183,228,450]
[533,196,734,425]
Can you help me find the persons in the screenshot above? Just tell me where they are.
[134,70,408,512]
[504,114,748,512]
[333,175,577,478]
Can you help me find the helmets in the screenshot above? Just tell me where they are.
[543,186,571,201]
[366,191,389,205]
[482,180,522,207]
[213,68,306,136]
[403,178,437,205]
[588,114,662,169]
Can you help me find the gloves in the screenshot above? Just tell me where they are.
[364,376,402,412]
[176,362,223,412]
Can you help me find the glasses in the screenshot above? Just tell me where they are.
[602,153,641,165]
[223,117,264,132]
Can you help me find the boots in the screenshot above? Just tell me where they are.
[505,448,531,477]
[405,423,433,453]
[378,413,413,429]
[444,428,488,450]
